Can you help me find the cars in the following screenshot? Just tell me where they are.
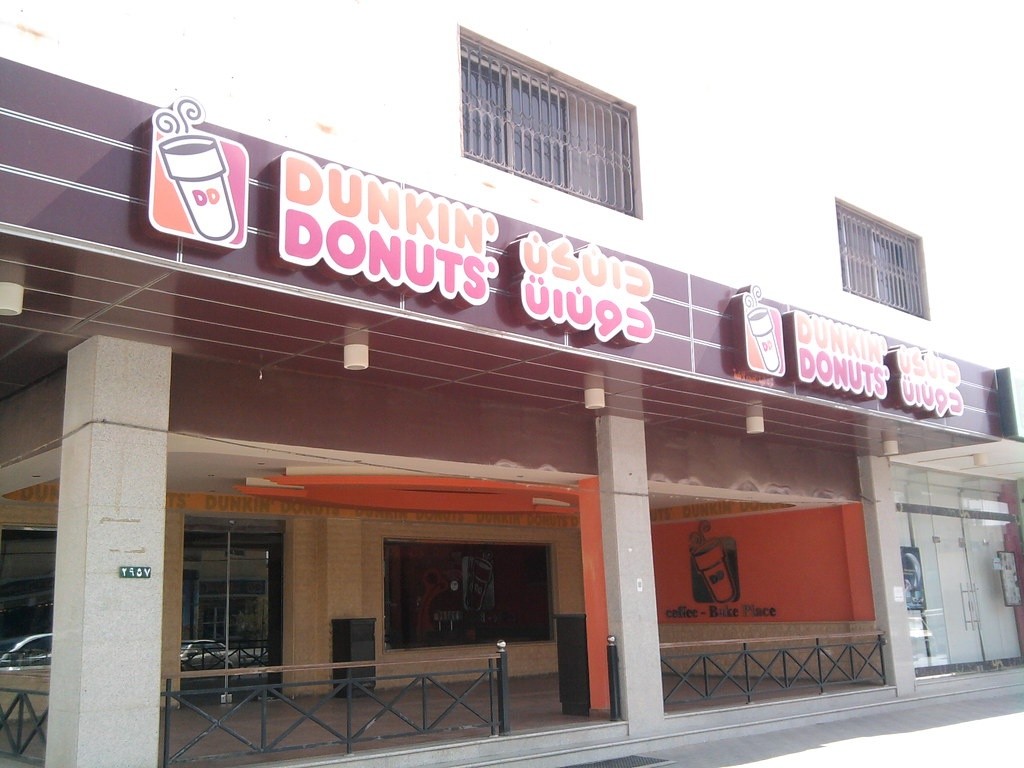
[1,632,51,666]
[180,639,234,671]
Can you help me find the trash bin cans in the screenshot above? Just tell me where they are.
[332,617,377,698]
[553,614,589,716]
